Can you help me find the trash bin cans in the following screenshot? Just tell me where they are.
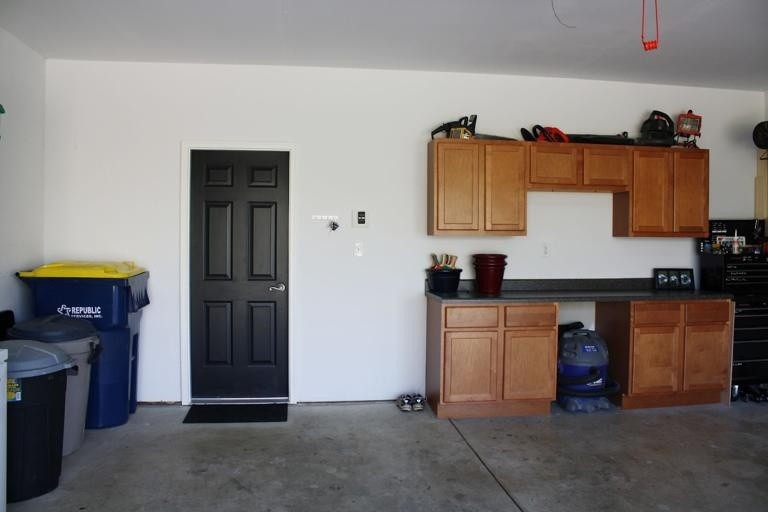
[6,315,103,457]
[14,261,150,430]
[0,341,78,503]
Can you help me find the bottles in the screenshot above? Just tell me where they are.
[703,241,711,252]
[732,229,739,255]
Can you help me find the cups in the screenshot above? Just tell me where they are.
[472,253,508,293]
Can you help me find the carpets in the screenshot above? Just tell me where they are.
[180,401,290,425]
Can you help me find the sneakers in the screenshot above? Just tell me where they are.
[394,393,425,414]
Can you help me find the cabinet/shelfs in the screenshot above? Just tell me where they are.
[593,294,731,407]
[524,140,627,194]
[425,135,524,239]
[611,144,710,239]
[422,297,559,422]
[696,218,766,404]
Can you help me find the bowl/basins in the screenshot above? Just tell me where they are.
[426,268,461,292]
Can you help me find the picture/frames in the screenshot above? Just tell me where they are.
[652,266,696,292]
